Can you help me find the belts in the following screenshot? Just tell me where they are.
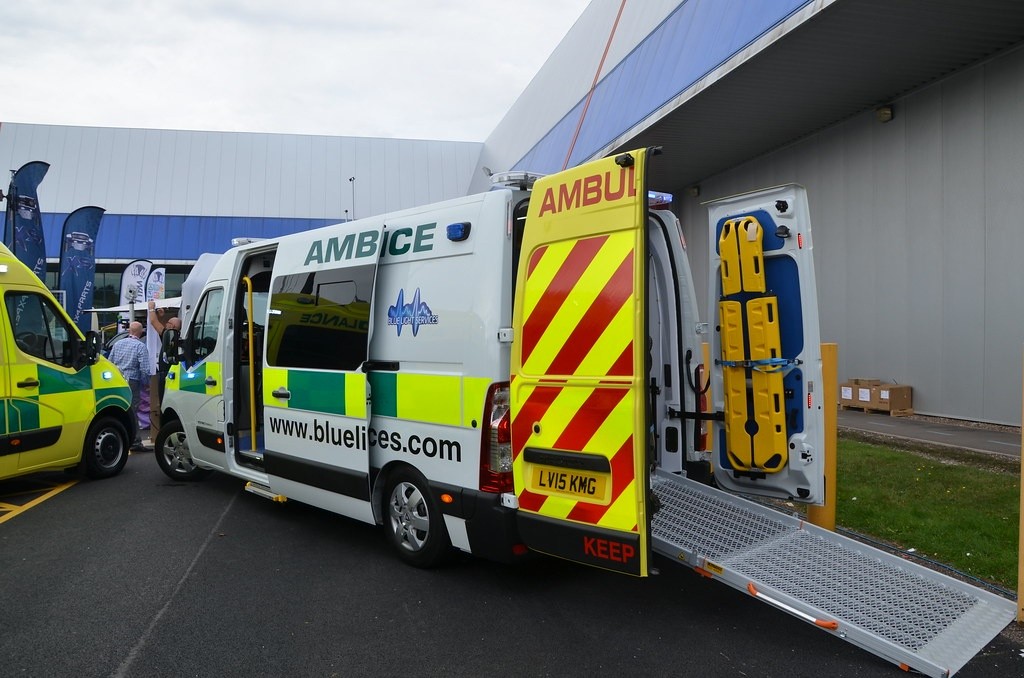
[129,378,140,382]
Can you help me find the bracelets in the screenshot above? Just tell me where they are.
[149,309,155,312]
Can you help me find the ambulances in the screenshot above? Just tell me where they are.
[1,241,137,480]
[154,145,826,577]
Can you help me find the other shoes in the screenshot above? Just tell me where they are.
[131,446,153,454]
[142,440,154,446]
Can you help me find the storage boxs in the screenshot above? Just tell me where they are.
[841,379,913,417]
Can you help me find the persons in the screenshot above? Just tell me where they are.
[147,301,183,408]
[108,321,154,452]
